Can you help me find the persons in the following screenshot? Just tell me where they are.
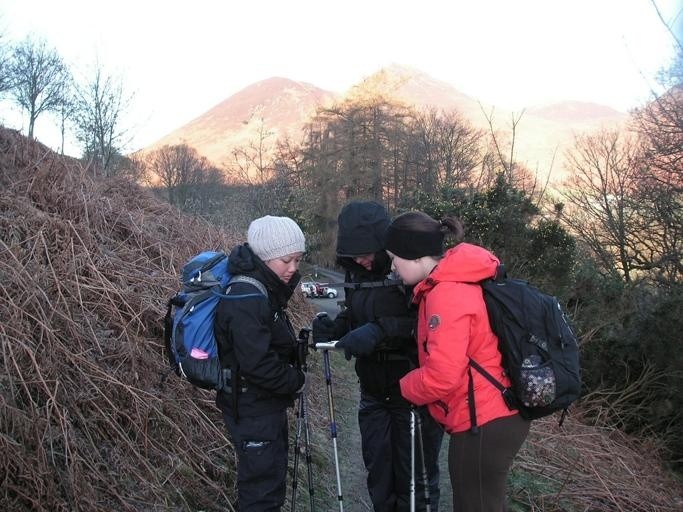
[307,199,445,511]
[374,211,533,511]
[214,213,312,512]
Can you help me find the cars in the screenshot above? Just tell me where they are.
[299,280,338,299]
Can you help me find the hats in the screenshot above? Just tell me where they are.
[246,215,307,261]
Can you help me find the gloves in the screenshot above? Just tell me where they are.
[312,318,344,342]
[338,323,383,361]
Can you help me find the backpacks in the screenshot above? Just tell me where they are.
[466,263,581,422]
[164,252,269,388]
